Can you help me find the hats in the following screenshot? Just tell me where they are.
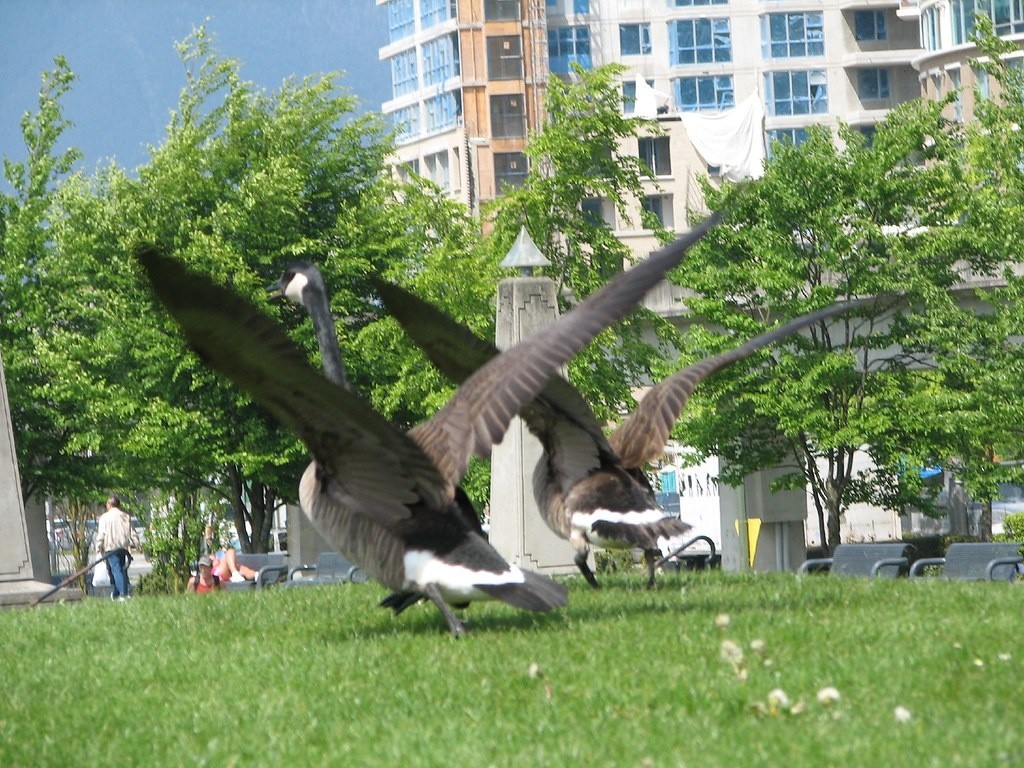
[198,557,212,566]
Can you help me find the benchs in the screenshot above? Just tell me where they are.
[910,542,1022,581]
[234,554,289,588]
[282,553,367,586]
[796,540,917,581]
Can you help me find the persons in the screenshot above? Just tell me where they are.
[95,496,259,603]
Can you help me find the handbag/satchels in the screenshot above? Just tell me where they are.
[92,552,110,588]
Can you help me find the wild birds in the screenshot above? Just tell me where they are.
[133,212,721,638]
[363,272,908,590]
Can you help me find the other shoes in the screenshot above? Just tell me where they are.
[128,596,132,599]
[117,597,125,601]
[231,572,245,582]
